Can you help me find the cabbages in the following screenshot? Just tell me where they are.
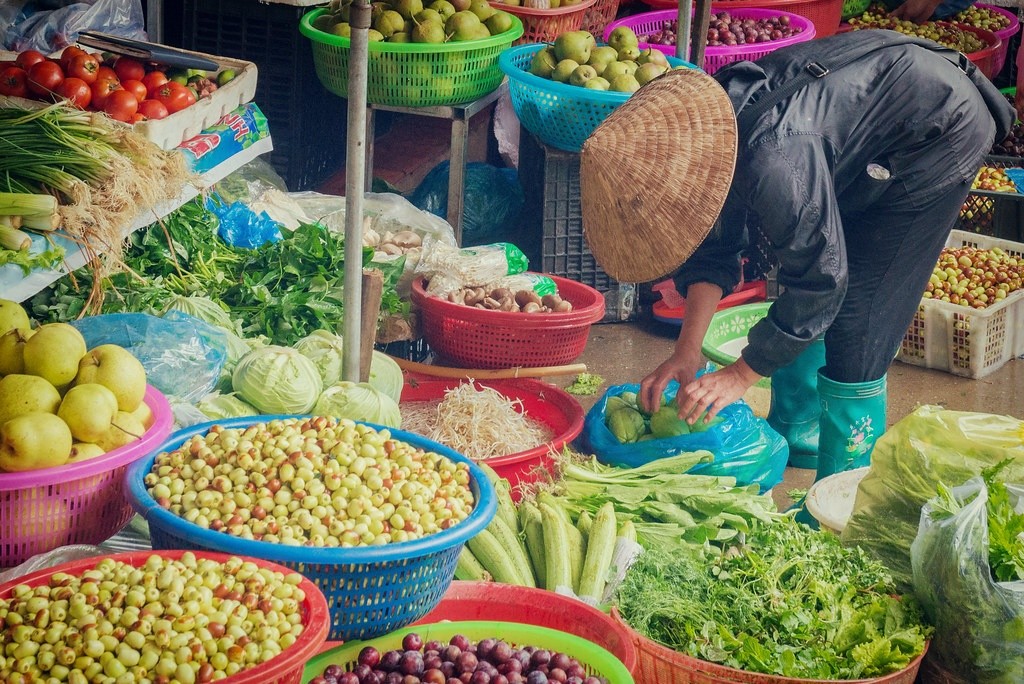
[164,329,403,431]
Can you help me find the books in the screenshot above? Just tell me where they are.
[75,31,220,72]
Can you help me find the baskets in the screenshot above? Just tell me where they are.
[301,0,1024,379]
[0,271,929,684]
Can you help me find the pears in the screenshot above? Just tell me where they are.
[0,298,156,565]
[311,0,689,107]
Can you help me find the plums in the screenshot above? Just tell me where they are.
[637,10,804,47]
[307,632,611,684]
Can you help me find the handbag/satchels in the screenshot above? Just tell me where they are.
[844,406,1024,684]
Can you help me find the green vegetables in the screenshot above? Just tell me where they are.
[0,189,411,347]
[518,405,1024,683]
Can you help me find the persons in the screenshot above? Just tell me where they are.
[887,0,1024,124]
[580,27,998,535]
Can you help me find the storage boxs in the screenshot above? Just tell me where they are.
[517,124,639,325]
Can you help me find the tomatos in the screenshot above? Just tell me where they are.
[0,44,196,125]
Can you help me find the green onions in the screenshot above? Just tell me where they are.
[0,92,210,240]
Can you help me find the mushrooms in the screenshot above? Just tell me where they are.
[449,286,573,313]
